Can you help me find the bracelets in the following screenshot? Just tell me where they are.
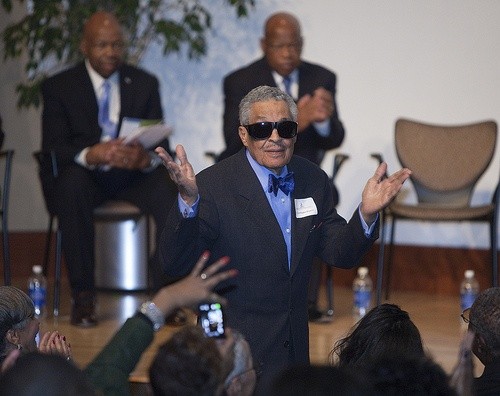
[140,300,165,332]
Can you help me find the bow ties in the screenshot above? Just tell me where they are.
[268,172,295,196]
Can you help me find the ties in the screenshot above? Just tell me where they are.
[284,77,291,94]
[97,80,114,138]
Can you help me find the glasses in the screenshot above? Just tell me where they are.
[243,121,298,138]
[461,307,480,330]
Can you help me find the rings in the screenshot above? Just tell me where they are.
[67,356,70,361]
[199,272,208,280]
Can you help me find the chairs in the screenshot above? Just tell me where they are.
[369,118,500,308]
[31,150,153,317]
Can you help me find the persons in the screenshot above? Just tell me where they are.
[40,12,177,328]
[149,85,411,396]
[222,12,345,322]
[0,251,500,396]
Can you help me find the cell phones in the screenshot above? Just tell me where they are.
[198,302,225,342]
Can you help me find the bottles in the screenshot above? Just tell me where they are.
[354,267,372,320]
[28,265,49,323]
[460,269,480,327]
